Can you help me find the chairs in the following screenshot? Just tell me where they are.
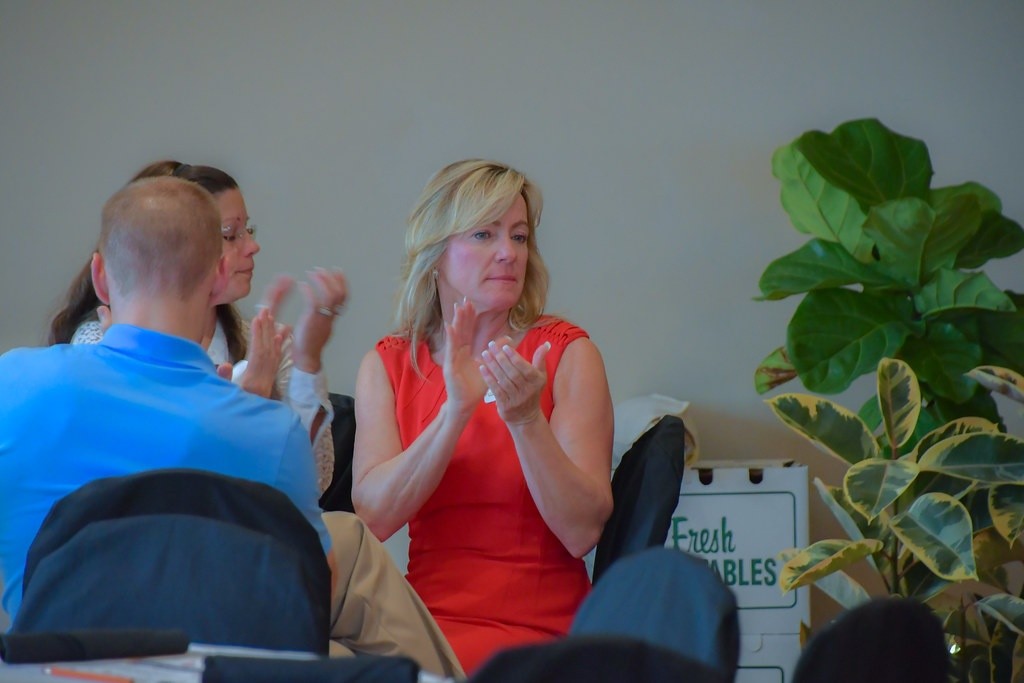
[569,545,742,676]
[583,413,687,588]
[793,597,948,683]
[10,466,332,657]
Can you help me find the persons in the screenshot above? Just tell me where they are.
[0,178,465,679]
[351,160,614,677]
[48,158,345,507]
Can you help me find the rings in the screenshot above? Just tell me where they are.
[319,307,334,317]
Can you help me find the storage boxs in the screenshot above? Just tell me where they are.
[733,630,802,683]
[661,456,811,636]
